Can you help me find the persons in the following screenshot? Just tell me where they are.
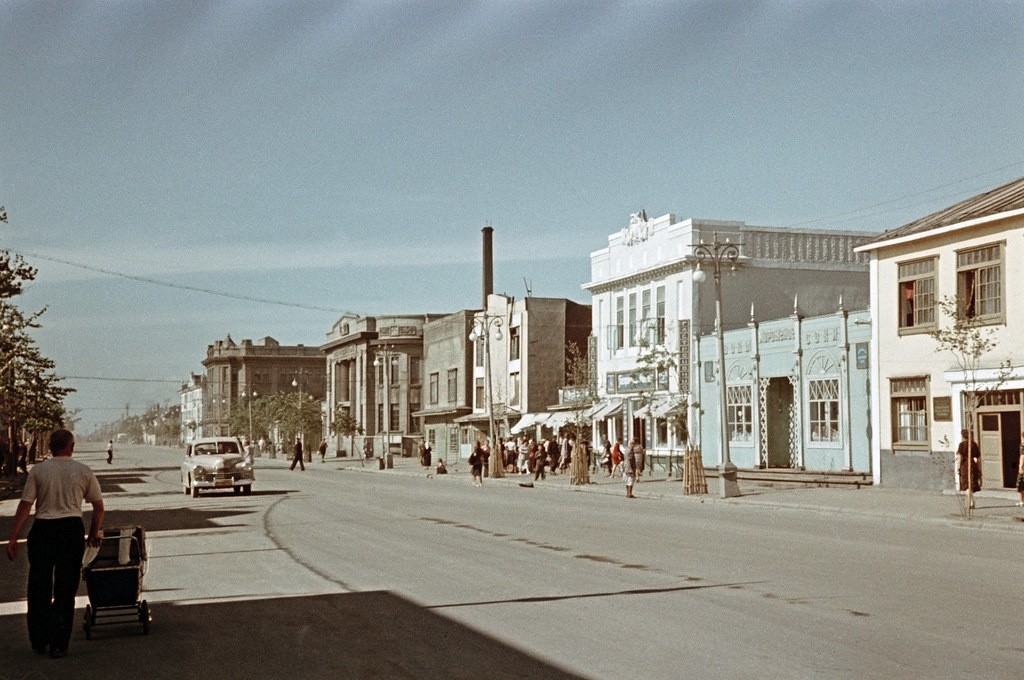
[252,433,272,454]
[288,438,305,471]
[436,459,447,474]
[600,433,613,477]
[318,438,328,463]
[5,428,105,658]
[610,437,625,478]
[363,440,370,463]
[106,440,113,464]
[418,437,433,470]
[468,431,592,487]
[1016,444,1024,508]
[956,429,982,510]
[237,438,254,470]
[623,437,644,497]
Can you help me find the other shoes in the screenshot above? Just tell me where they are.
[626,494,633,499]
[36,644,47,655]
[49,649,67,659]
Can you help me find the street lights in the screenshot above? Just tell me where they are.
[289,366,313,438]
[468,307,507,478]
[372,341,399,468]
[686,232,750,499]
[240,383,258,444]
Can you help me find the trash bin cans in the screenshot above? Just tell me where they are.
[254,445,260,457]
[380,457,385,469]
[269,445,276,459]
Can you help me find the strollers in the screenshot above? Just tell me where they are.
[83,522,152,639]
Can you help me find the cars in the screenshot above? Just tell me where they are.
[179,436,255,498]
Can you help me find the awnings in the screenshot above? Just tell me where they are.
[632,394,688,419]
[509,412,551,435]
[544,409,593,429]
[590,399,622,423]
